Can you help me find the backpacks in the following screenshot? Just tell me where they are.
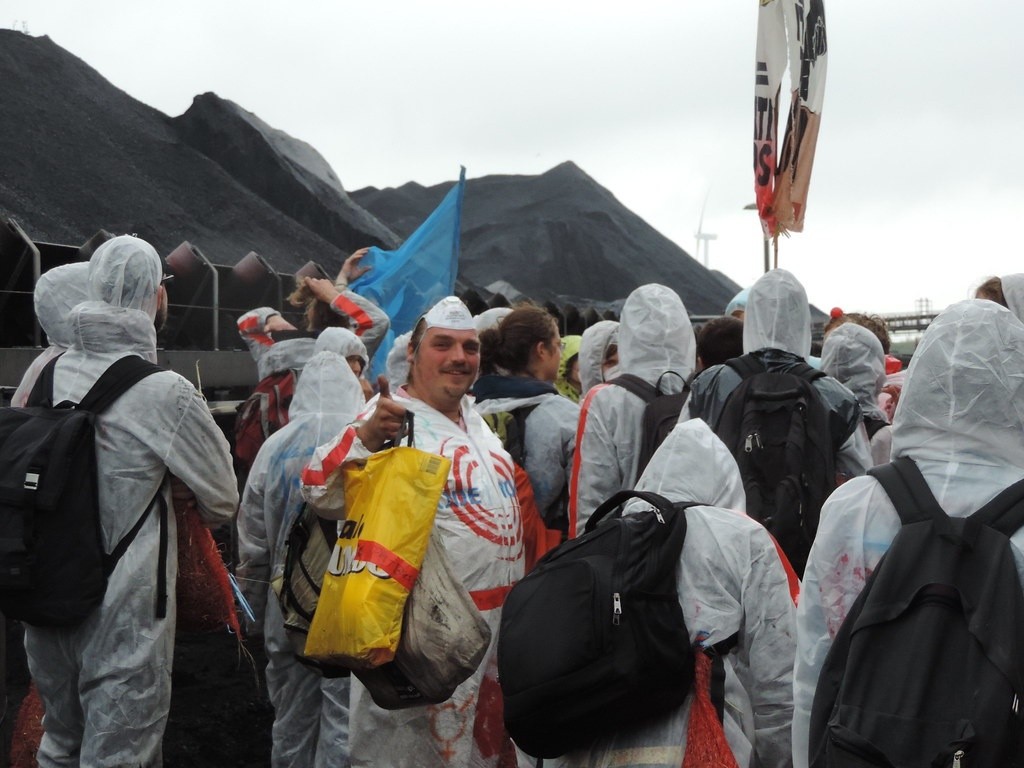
[714,360,836,560]
[482,402,536,466]
[0,351,172,629]
[807,456,1024,768]
[496,491,742,759]
[609,374,691,485]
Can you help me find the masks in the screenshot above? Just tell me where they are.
[414,295,479,356]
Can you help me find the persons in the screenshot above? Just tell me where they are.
[17,235,242,768]
[972,270,1024,322]
[789,294,1024,768]
[235,246,933,767]
[9,261,167,410]
[299,294,550,768]
[504,417,803,766]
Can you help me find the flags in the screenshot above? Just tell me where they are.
[343,178,462,387]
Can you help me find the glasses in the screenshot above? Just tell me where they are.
[160,275,178,292]
[545,341,567,352]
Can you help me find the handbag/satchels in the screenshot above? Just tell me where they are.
[282,408,495,712]
